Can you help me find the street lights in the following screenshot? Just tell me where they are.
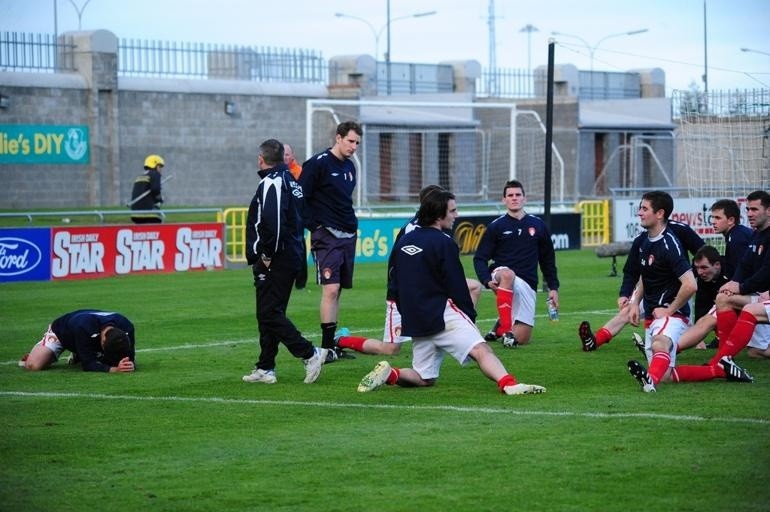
[552,29,651,99]
[335,9,440,94]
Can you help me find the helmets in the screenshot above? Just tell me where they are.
[143,153,165,170]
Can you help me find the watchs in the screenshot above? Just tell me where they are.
[261,253,271,262]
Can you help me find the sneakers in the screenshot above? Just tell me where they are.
[717,354,755,385]
[241,368,279,384]
[300,346,330,385]
[333,327,351,349]
[627,359,657,394]
[330,346,357,360]
[631,332,648,361]
[578,320,597,353]
[18,352,30,367]
[357,360,393,394]
[501,331,519,349]
[502,382,547,396]
[484,318,502,342]
[67,352,79,365]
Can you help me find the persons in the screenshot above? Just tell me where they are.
[243,139,328,384]
[474,180,560,346]
[692,242,729,351]
[358,189,547,396]
[18,308,136,372]
[703,190,770,366]
[617,190,754,392]
[331,186,482,355]
[297,121,363,359]
[130,155,167,223]
[578,201,707,352]
[631,199,752,361]
[282,144,308,289]
